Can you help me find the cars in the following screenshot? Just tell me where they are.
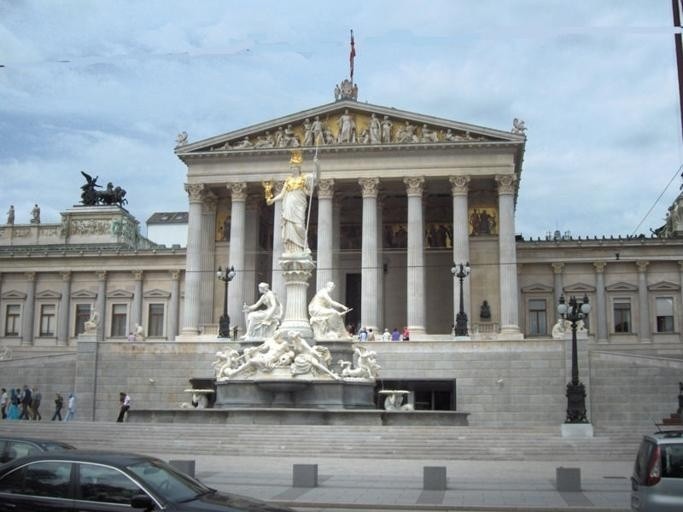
[0,437,295,511]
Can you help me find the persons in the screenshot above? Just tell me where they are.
[552,318,564,340]
[385,394,413,411]
[116,392,130,422]
[63,393,75,422]
[266,151,320,259]
[6,205,15,225]
[50,393,63,420]
[310,209,501,250]
[348,323,410,341]
[88,177,102,190]
[662,212,672,238]
[215,279,381,379]
[31,204,41,219]
[0,385,41,421]
[218,108,480,146]
[672,202,681,225]
[181,395,207,408]
[334,79,360,101]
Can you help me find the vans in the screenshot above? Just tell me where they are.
[631,430,683,512]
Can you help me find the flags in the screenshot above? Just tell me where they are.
[349,31,357,79]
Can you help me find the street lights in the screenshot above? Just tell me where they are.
[451,262,471,336]
[216,266,236,337]
[557,293,591,422]
